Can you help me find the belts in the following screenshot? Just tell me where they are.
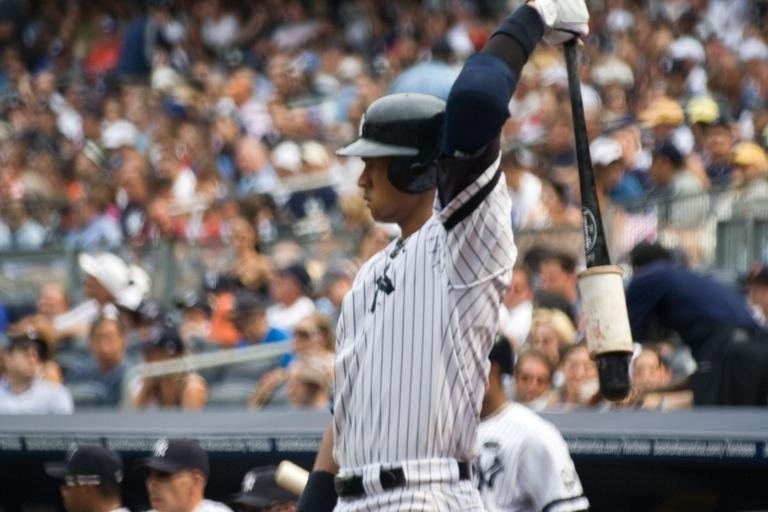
[334,464,469,499]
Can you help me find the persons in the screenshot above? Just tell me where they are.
[0,0,768,512]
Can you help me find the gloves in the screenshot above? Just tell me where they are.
[526,0,590,50]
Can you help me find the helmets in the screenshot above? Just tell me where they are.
[335,92,448,194]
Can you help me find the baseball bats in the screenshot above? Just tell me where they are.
[562,38,633,399]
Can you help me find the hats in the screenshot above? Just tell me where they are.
[231,466,298,509]
[45,444,127,487]
[134,438,213,479]
